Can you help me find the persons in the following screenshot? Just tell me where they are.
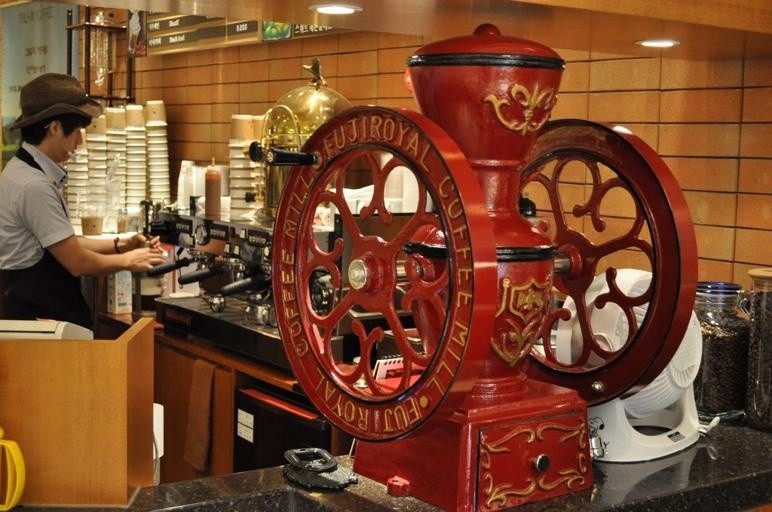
[0,73,168,329]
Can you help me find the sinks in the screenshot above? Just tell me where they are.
[150,487,334,512]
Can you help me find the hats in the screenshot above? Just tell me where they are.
[9,73,103,132]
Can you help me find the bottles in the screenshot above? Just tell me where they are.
[204,157,221,221]
[695,266,771,431]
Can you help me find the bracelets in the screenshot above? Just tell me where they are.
[114,237,120,254]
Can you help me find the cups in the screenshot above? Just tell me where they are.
[388,198,402,213]
[62,99,195,235]
[229,115,264,224]
